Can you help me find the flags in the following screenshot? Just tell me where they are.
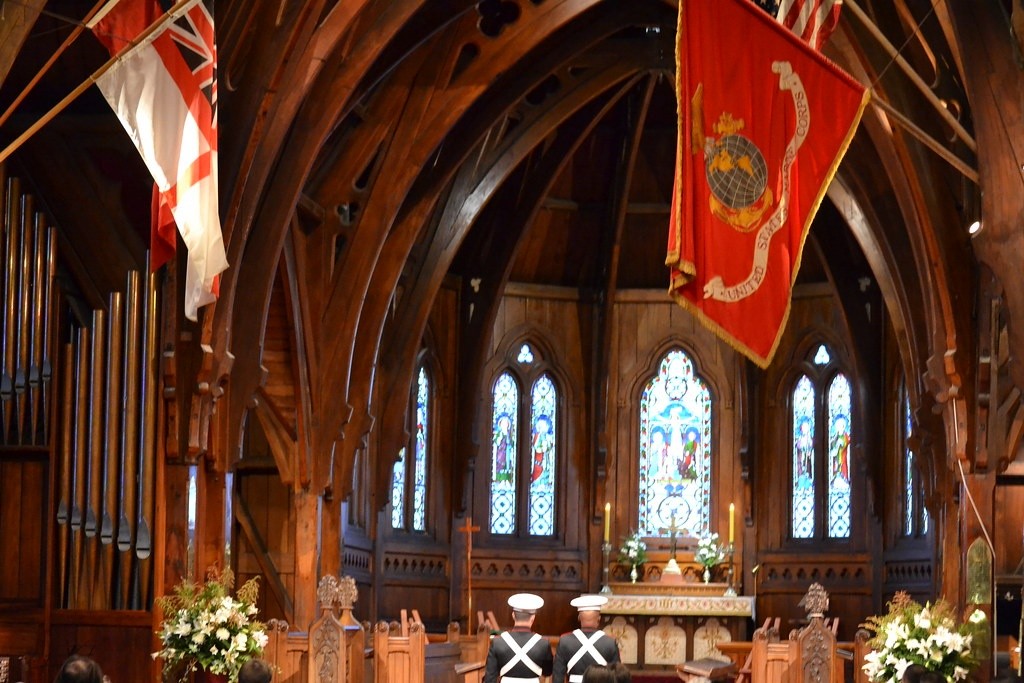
[774,0,843,53]
[86,0,231,323]
[666,0,872,370]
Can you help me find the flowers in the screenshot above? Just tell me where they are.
[693,532,729,568]
[150,561,281,683]
[616,527,648,567]
[858,591,987,683]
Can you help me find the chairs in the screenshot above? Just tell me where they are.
[674,617,780,683]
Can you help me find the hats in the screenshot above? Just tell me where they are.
[571,595,607,611]
[508,593,544,614]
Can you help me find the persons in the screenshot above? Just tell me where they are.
[55,655,104,683]
[483,593,554,683]
[552,595,621,683]
[238,659,272,683]
[582,661,632,683]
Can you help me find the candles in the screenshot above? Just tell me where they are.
[729,503,734,542]
[604,502,610,542]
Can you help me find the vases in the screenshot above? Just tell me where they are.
[703,566,710,584]
[631,564,638,584]
